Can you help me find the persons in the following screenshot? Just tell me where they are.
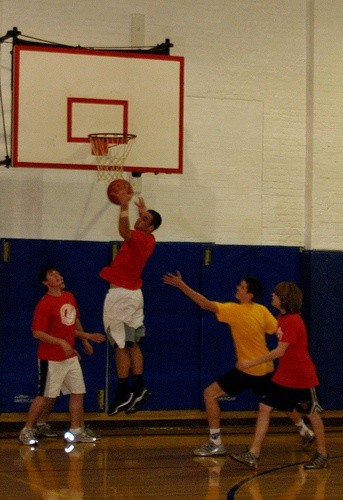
[18,266,105,446]
[98,183,162,416]
[228,282,332,469]
[164,270,316,457]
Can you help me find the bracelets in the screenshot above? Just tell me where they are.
[120,212,129,217]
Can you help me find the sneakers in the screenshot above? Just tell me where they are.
[65,430,96,443]
[107,389,134,416]
[19,427,39,445]
[231,452,258,466]
[85,424,102,440]
[193,440,226,456]
[300,430,314,451]
[125,385,152,414]
[304,452,329,469]
[36,423,57,437]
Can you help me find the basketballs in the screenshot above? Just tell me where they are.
[108,179,132,205]
